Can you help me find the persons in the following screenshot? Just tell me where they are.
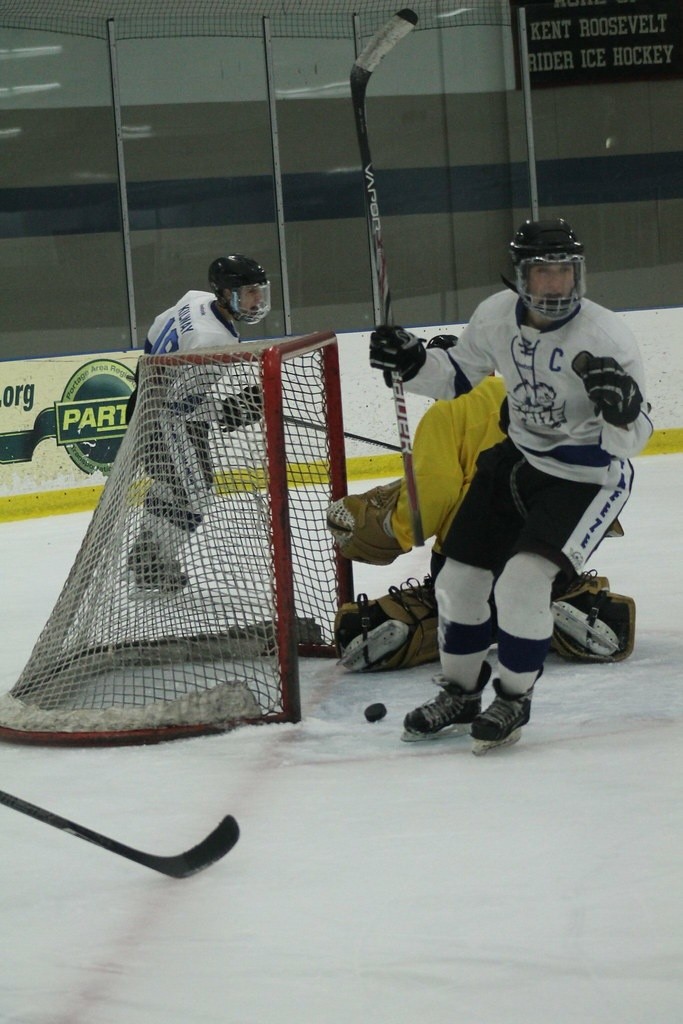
[121,254,271,600]
[326,217,654,757]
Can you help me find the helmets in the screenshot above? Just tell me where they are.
[511,217,587,265]
[208,253,271,291]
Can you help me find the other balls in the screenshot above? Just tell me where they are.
[363,702,388,723]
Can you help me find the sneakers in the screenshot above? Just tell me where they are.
[132,532,185,590]
[472,667,545,756]
[402,659,492,740]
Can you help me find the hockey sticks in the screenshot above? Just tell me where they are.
[348,6,428,548]
[282,413,405,454]
[0,787,241,880]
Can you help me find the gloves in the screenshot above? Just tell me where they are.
[225,388,261,431]
[585,355,643,426]
[370,325,428,386]
